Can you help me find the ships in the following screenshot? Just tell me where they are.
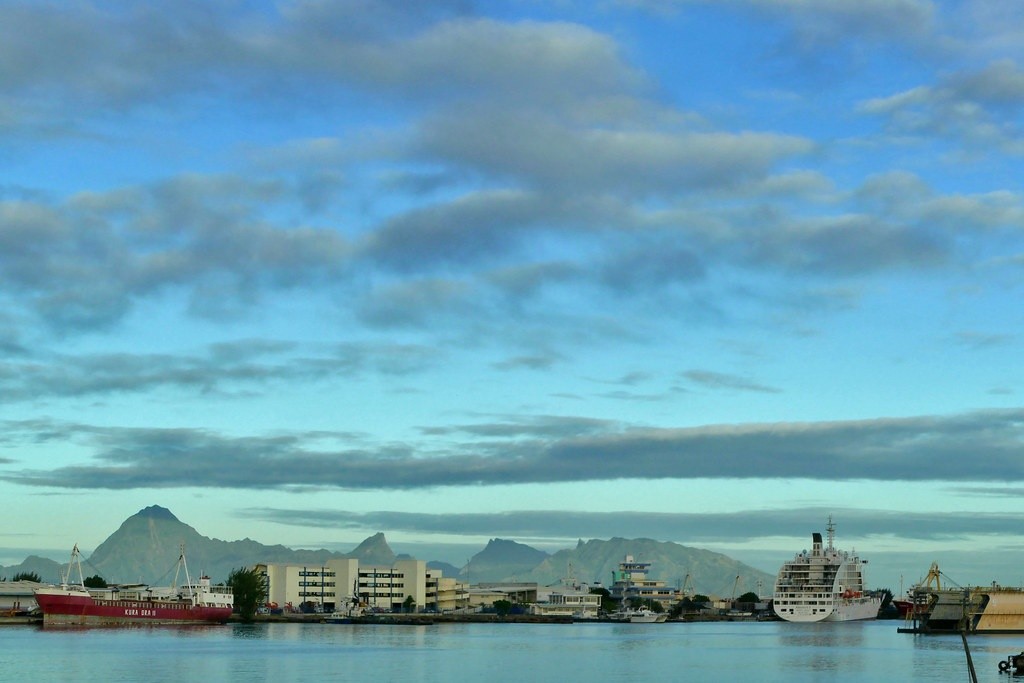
[772,513,886,623]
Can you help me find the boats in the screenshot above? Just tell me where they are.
[572,605,671,623]
[33,542,234,623]
[324,608,362,625]
[892,562,942,616]
